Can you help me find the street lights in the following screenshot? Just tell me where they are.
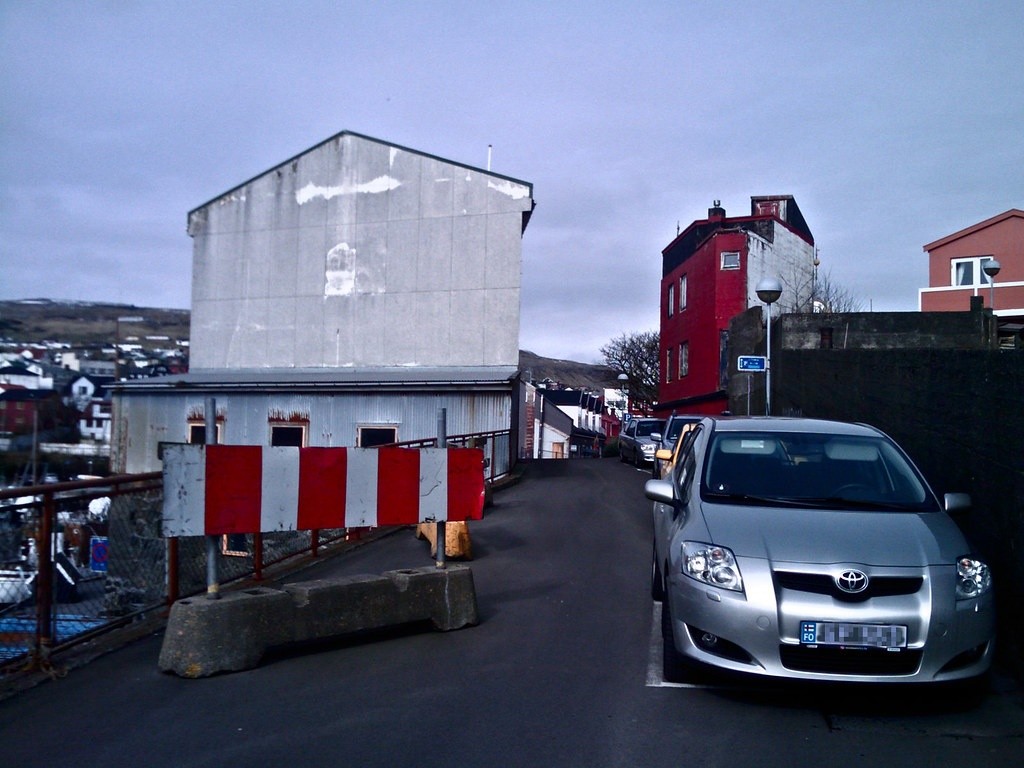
[754,277,782,417]
[617,373,630,430]
[984,260,1001,315]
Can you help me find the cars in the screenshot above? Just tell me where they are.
[643,415,1000,706]
[655,423,697,481]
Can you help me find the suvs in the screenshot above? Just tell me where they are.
[651,408,737,481]
[618,418,667,469]
